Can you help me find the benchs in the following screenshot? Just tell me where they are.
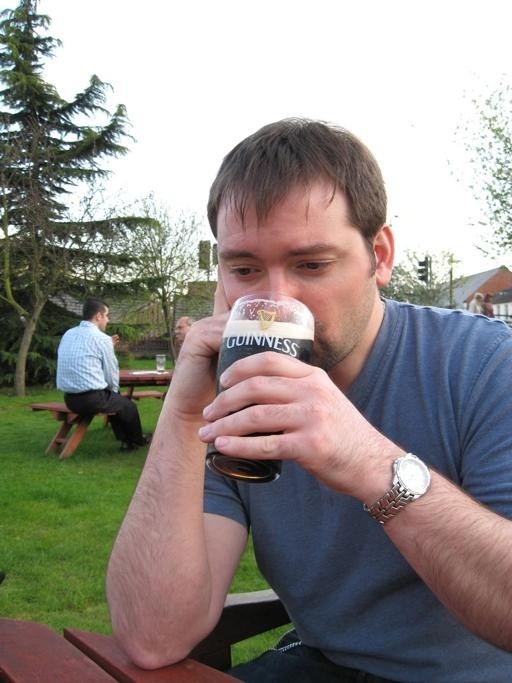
[194,590,286,667]
[33,403,115,459]
[121,392,165,401]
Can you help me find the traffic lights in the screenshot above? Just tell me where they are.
[418,255,432,285]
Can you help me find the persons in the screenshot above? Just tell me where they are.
[56,298,152,451]
[469,292,494,318]
[105,118,512,683]
[175,316,195,342]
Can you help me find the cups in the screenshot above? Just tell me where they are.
[156,354,165,372]
[204,291,317,482]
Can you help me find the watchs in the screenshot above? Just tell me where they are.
[363,453,431,528]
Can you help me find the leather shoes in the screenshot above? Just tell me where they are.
[120,434,151,454]
